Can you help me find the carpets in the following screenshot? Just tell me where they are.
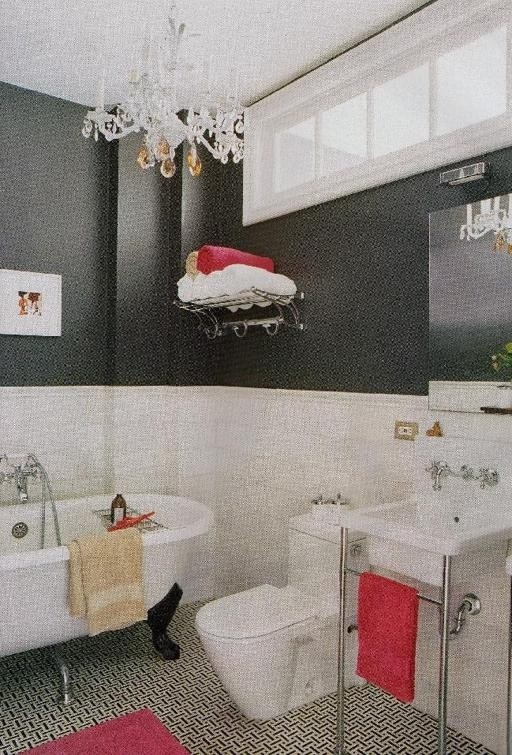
[16,701,188,754]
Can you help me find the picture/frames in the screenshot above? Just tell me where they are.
[0,267,64,340]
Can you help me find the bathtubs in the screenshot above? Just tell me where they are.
[1,493,212,658]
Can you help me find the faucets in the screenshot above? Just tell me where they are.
[431,464,474,492]
[12,465,30,505]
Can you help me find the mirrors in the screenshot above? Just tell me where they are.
[425,190,512,384]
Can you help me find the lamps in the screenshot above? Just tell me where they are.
[456,198,511,251]
[77,5,248,176]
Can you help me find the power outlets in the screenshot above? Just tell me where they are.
[392,419,418,442]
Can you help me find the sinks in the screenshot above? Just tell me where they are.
[335,499,512,587]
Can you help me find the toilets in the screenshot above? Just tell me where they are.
[193,513,368,721]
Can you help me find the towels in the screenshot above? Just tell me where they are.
[173,240,296,303]
[67,525,150,639]
[352,572,420,709]
